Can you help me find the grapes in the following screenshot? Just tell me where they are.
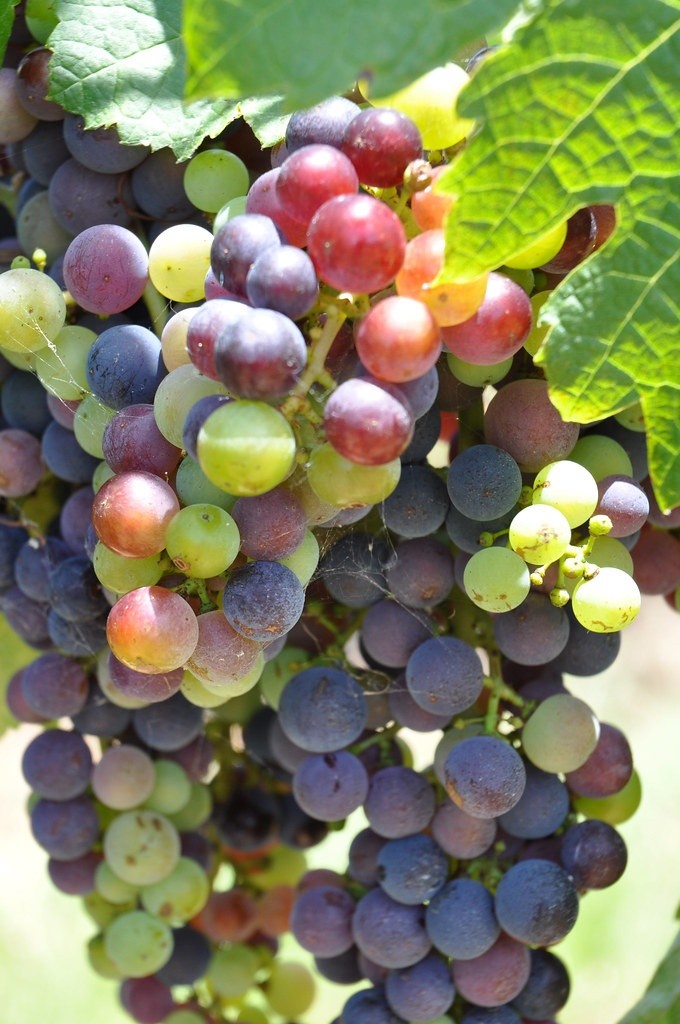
[0,0,680,1024]
